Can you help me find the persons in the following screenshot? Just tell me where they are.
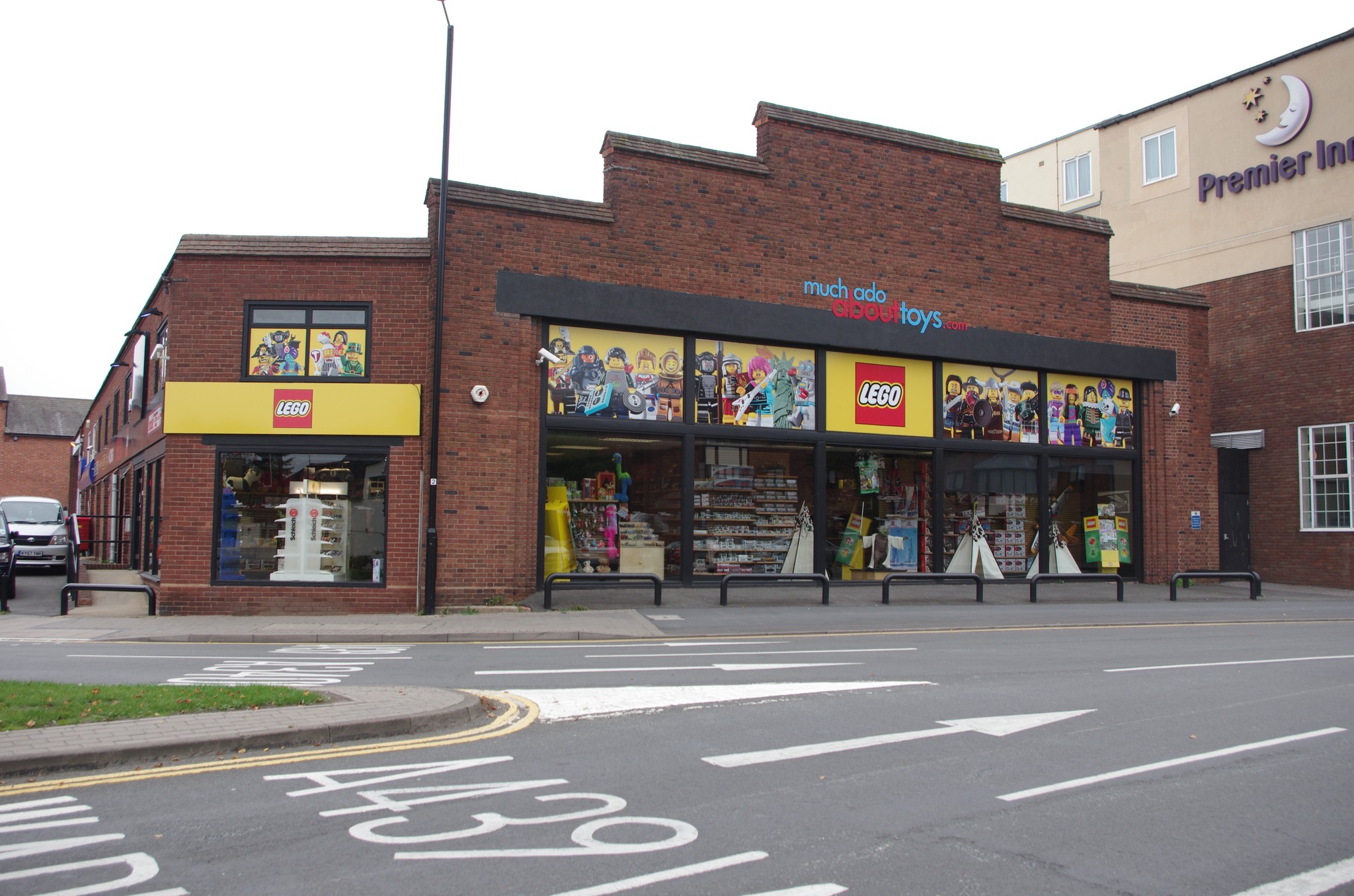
[858,525,908,569]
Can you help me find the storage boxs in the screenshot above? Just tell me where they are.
[693,509,756,520]
[756,502,797,512]
[942,554,954,572]
[943,498,955,517]
[756,514,797,525]
[920,498,933,517]
[1000,490,1028,575]
[942,517,955,534]
[920,516,933,534]
[547,475,598,500]
[694,553,787,573]
[693,523,755,535]
[692,493,755,507]
[943,535,955,553]
[694,463,754,490]
[920,461,934,481]
[920,554,933,573]
[754,478,799,488]
[1080,503,1133,575]
[920,535,933,552]
[692,538,792,550]
[755,490,799,501]
[959,491,991,534]
[920,481,933,498]
[755,527,796,535]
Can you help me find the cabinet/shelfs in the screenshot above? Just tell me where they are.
[754,473,800,574]
[958,494,995,555]
[712,485,756,578]
[220,492,352,582]
[618,519,666,584]
[994,502,1030,575]
[630,486,682,579]
[880,462,960,574]
[691,487,714,577]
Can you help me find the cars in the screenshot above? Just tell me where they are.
[0,495,71,575]
[0,507,20,600]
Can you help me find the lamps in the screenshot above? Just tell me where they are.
[139,307,163,319]
[123,328,144,336]
[109,360,130,368]
[70,440,84,447]
